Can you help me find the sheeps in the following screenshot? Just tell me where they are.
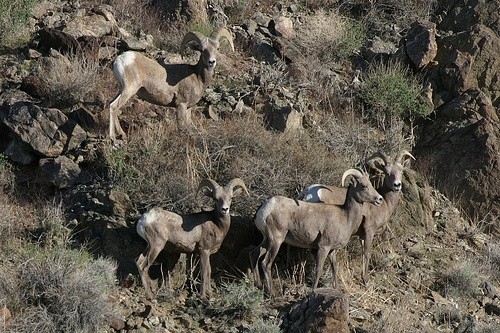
[295,149,416,287]
[134,178,249,303]
[252,166,384,300]
[108,27,235,142]
